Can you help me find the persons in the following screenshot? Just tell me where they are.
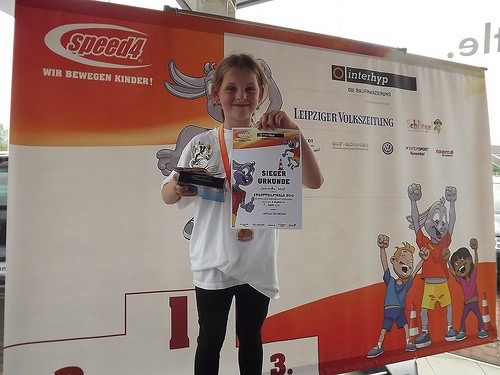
[161,53,324,375]
